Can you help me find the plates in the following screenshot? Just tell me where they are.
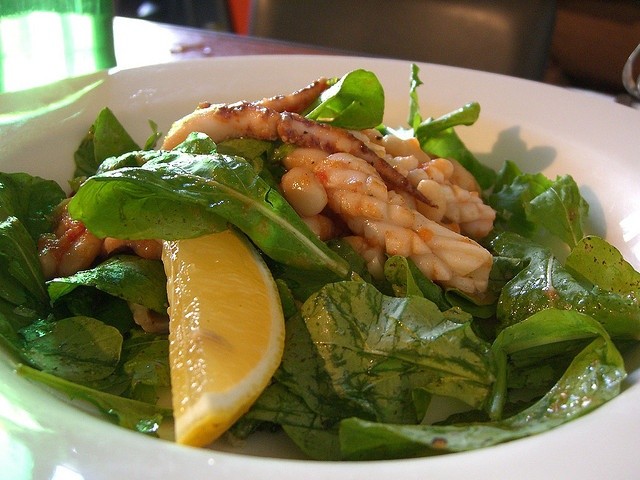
[1,51,640,480]
